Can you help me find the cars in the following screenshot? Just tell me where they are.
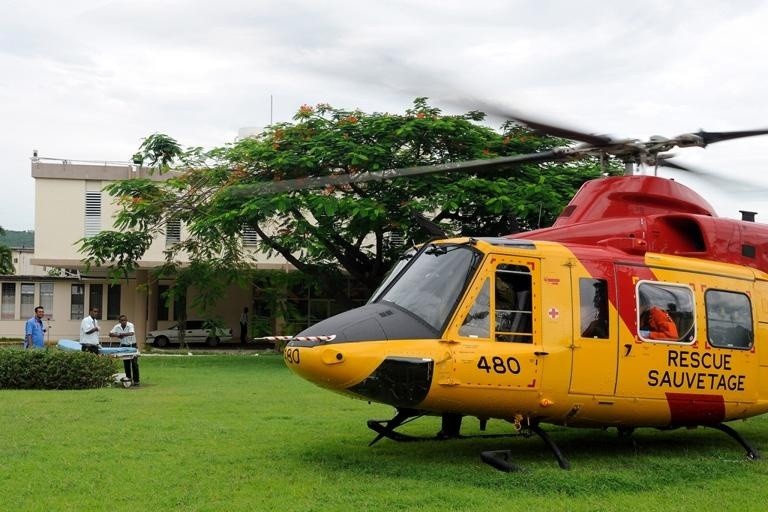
[145,318,232,348]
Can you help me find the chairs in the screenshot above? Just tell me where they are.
[507,290,534,342]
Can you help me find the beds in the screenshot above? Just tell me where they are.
[56,338,140,389]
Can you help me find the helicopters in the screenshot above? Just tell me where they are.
[219,98,768,473]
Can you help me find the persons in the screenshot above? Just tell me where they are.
[239,305,250,345]
[639,293,679,341]
[79,304,103,355]
[23,306,52,350]
[108,314,140,387]
